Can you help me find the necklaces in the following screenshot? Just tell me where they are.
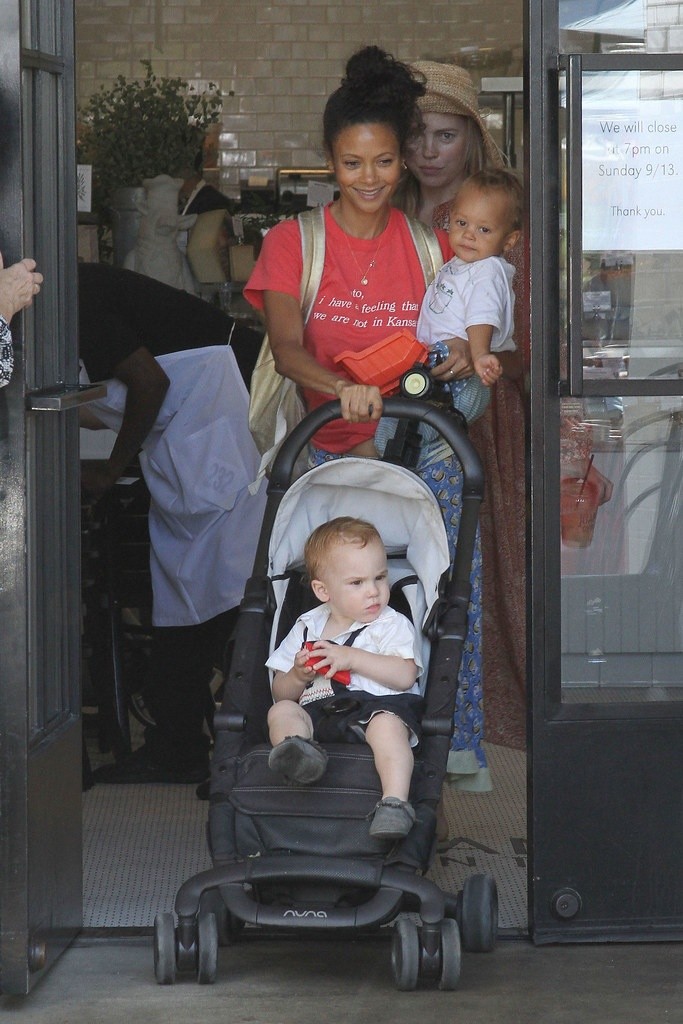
[342,226,382,286]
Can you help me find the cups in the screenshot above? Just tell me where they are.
[561,476,601,547]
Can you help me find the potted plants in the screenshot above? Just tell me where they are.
[76,57,236,263]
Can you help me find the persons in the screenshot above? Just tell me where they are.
[0,253,44,389]
[374,168,521,458]
[243,46,491,790]
[176,127,239,215]
[394,61,614,750]
[70,260,262,786]
[263,517,427,838]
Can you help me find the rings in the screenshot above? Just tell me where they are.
[449,369,455,377]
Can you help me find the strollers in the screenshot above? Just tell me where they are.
[153,371,505,994]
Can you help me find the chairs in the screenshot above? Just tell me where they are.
[100,461,219,781]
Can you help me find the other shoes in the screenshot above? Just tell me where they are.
[269,736,327,783]
[107,726,212,784]
[366,797,422,837]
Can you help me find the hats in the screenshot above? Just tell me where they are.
[407,61,507,171]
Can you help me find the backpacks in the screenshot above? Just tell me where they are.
[247,207,443,485]
